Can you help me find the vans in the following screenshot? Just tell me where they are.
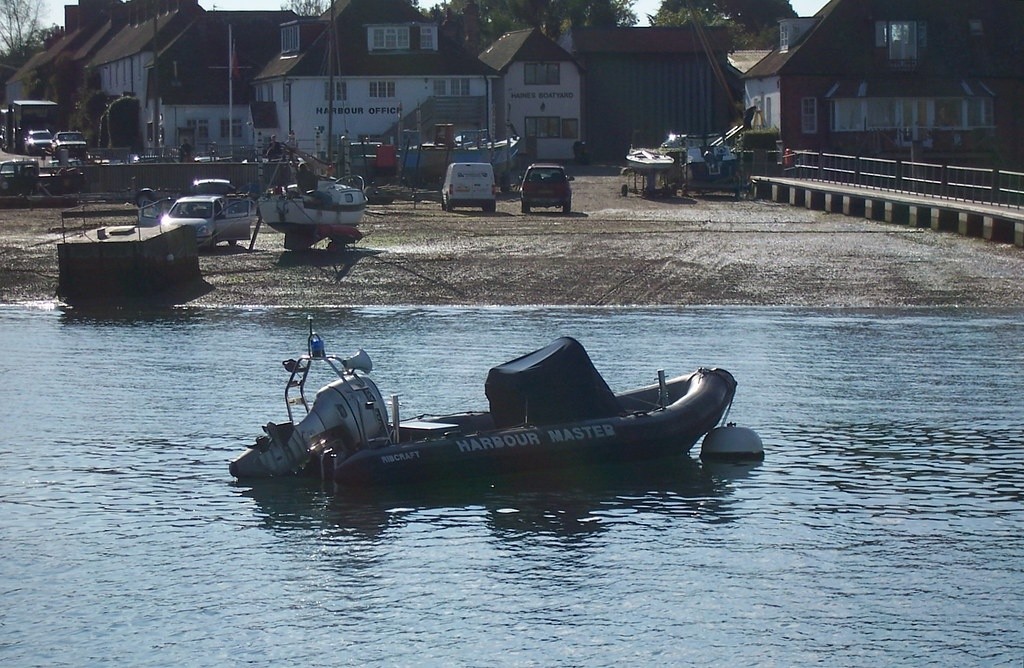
[139,196,251,249]
[442,162,498,212]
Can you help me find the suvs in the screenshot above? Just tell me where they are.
[24,129,54,155]
[519,163,575,212]
[52,130,86,158]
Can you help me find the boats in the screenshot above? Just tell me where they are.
[681,146,739,190]
[625,145,675,171]
[228,314,738,492]
[395,135,521,183]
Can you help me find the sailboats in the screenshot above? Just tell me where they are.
[256,0,367,252]
